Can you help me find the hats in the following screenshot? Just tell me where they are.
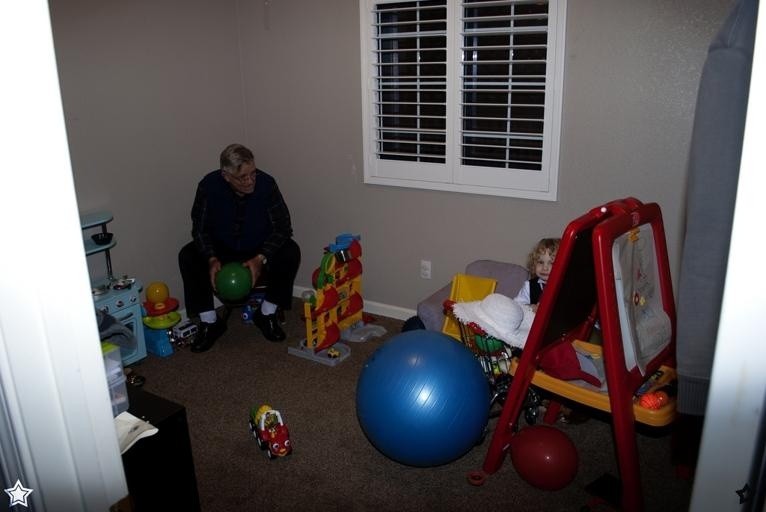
[451,294,537,350]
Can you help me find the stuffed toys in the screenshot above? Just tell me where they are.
[288,232,387,368]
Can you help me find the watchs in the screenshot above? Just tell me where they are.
[258,253,267,265]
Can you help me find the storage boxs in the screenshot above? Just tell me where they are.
[101,341,130,416]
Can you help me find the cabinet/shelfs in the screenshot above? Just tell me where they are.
[121,380,201,511]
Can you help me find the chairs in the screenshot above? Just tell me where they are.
[401,259,533,386]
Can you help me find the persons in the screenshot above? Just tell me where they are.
[514,238,562,313]
[177,142,302,353]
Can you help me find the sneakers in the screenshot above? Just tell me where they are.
[251,304,286,343]
[190,315,228,353]
